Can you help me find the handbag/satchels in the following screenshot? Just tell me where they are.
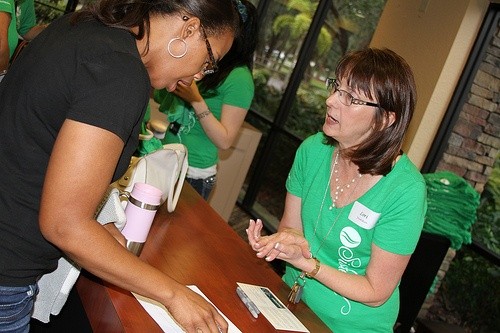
[121,143,188,213]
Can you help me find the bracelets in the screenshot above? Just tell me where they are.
[197,109,210,118]
[310,258,320,276]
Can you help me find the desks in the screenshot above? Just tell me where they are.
[76,181,333,333]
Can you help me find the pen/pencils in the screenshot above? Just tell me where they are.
[235,287,261,318]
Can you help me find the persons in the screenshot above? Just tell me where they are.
[0,0,49,73]
[0,0,239,333]
[246,49,428,333]
[154,0,259,198]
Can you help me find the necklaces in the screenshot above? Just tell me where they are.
[311,151,361,257]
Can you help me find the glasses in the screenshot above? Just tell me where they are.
[183,16,219,76]
[326,78,380,108]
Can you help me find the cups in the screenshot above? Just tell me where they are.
[119,183,162,259]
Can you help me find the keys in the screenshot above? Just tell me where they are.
[288,284,300,304]
